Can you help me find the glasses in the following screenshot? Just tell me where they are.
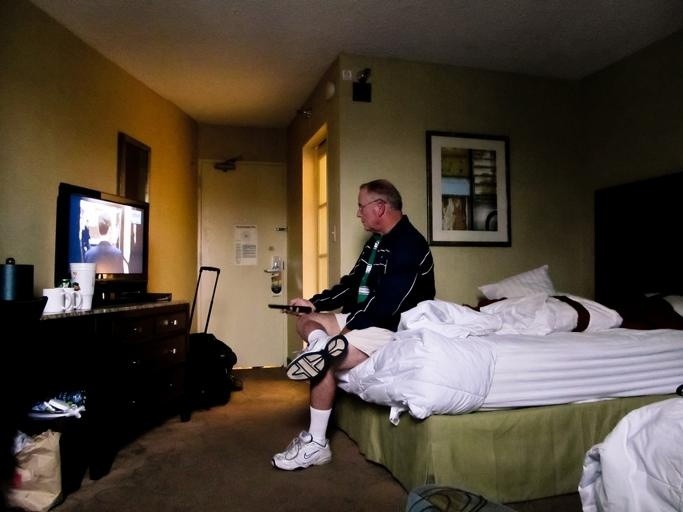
[358,198,387,211]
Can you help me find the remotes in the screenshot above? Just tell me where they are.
[268,304,312,314]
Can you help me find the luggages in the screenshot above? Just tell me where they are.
[179,262,238,407]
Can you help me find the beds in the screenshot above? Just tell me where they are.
[330,170,682,506]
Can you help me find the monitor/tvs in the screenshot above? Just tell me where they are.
[55,183,149,307]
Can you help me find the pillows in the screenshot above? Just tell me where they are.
[477,264,556,301]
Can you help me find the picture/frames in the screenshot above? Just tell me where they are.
[426,129,512,248]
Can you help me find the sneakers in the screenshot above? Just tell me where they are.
[271,431,332,472]
[285,333,349,382]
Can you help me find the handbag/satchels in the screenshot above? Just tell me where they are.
[4,428,63,512]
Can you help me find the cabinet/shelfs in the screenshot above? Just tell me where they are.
[0,300,190,478]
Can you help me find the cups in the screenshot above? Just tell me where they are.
[70,263,96,311]
[44,289,82,313]
[5,257,14,301]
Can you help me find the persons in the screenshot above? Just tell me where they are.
[83,218,91,252]
[268,176,436,472]
[83,209,125,275]
